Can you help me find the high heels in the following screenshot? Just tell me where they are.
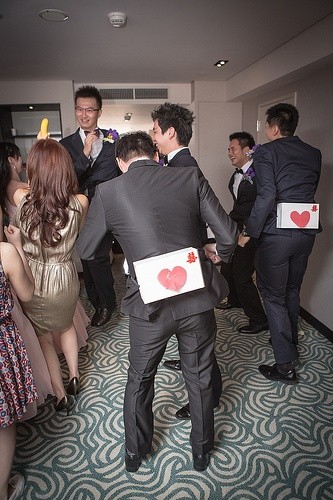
[54,395,74,416]
[67,377,80,399]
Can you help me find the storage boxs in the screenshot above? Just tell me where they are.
[276,202,320,229]
[133,247,206,305]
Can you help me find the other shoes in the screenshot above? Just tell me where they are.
[8,472,24,500]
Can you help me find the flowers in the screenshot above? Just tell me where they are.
[240,167,256,184]
[102,127,119,143]
[158,159,168,167]
[245,143,262,159]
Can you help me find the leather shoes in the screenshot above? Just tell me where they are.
[163,360,182,370]
[215,301,243,309]
[125,448,141,472]
[237,322,269,333]
[193,451,209,470]
[175,402,192,420]
[259,364,298,385]
[91,299,117,327]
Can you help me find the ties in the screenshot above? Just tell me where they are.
[83,131,91,138]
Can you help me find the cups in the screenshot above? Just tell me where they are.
[11,129,16,136]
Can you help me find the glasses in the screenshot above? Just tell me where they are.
[74,106,99,113]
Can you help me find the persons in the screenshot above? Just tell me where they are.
[0,142,30,227]
[237,104,321,385]
[216,132,268,334]
[59,86,123,328]
[78,131,239,472]
[151,103,222,419]
[0,226,38,500]
[14,140,90,417]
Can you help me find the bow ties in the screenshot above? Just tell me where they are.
[235,168,243,175]
[163,154,168,164]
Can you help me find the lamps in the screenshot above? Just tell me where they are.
[124,116,131,121]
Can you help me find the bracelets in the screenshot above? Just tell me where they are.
[241,229,250,237]
